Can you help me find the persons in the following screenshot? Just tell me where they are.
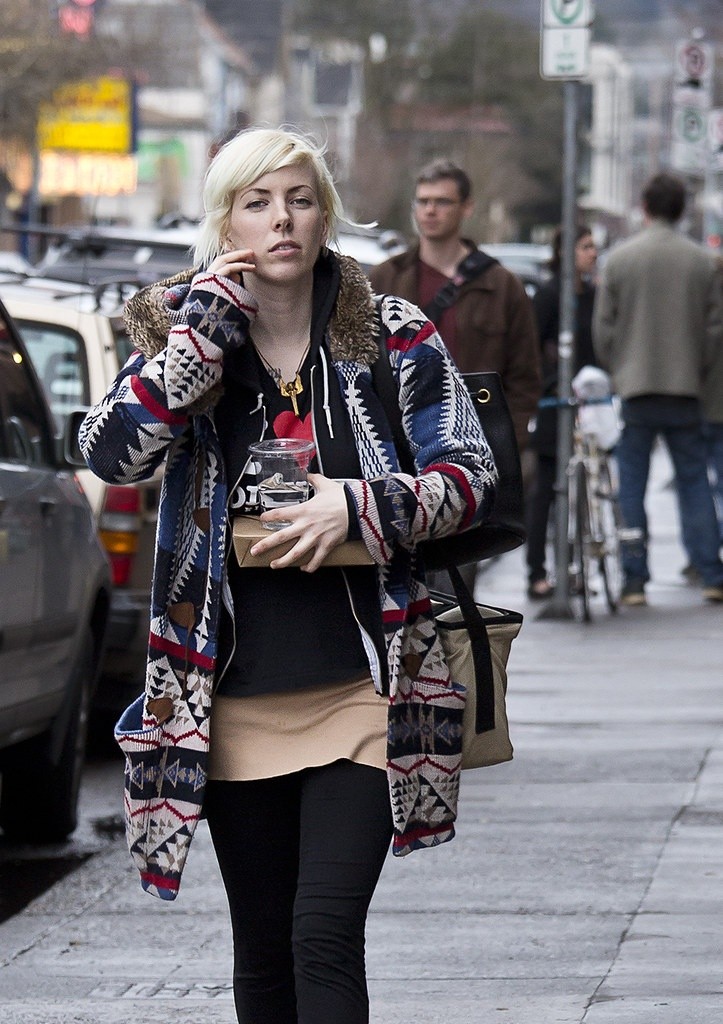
[366,160,545,604]
[592,170,723,607]
[523,221,600,598]
[78,127,501,1024]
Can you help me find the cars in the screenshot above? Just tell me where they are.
[1,300,113,835]
[0,232,213,684]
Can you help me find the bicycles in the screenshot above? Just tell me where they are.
[535,397,644,623]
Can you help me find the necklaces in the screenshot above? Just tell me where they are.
[251,337,310,416]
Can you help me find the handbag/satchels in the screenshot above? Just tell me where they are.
[428,589,523,771]
[374,294,526,573]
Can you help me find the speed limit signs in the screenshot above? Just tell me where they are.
[672,39,714,180]
[539,1,594,82]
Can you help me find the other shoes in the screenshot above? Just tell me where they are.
[703,587,723,601]
[619,591,644,605]
[526,584,554,600]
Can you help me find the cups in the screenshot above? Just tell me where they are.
[248,439,317,531]
